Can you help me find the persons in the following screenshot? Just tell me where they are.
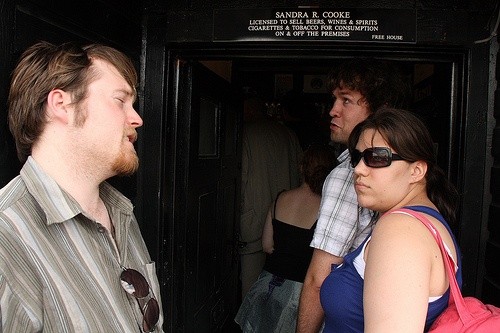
[0,39,164,333]
[295,57,420,333]
[234,148,335,333]
[319,107,463,333]
[228,91,304,303]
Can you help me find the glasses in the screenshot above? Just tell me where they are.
[351,147,405,168]
[120,265,160,332]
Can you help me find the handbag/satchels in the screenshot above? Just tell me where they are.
[380,208,500,333]
[234,270,326,333]
